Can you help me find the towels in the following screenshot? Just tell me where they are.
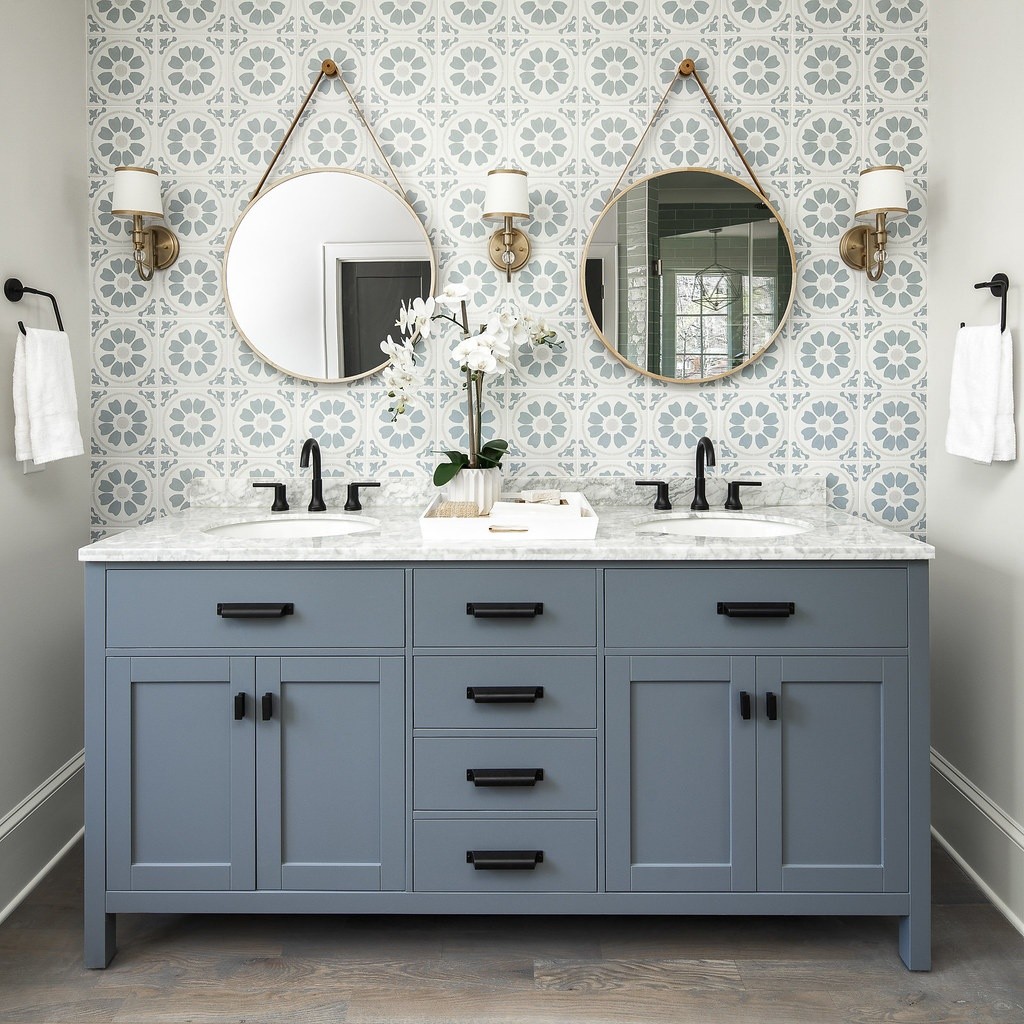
[946,322,1017,465]
[11,326,84,472]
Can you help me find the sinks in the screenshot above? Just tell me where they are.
[633,510,813,542]
[202,514,382,543]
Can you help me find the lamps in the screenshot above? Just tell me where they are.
[111,166,179,282]
[838,165,909,282]
[482,168,531,284]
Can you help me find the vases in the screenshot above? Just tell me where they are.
[447,465,501,515]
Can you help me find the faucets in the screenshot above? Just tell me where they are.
[300,435,325,511]
[691,436,717,509]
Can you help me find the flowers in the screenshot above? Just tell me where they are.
[379,284,568,489]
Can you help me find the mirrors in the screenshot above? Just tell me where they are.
[580,58,798,384]
[222,59,436,386]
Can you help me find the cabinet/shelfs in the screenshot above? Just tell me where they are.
[85,560,932,972]
